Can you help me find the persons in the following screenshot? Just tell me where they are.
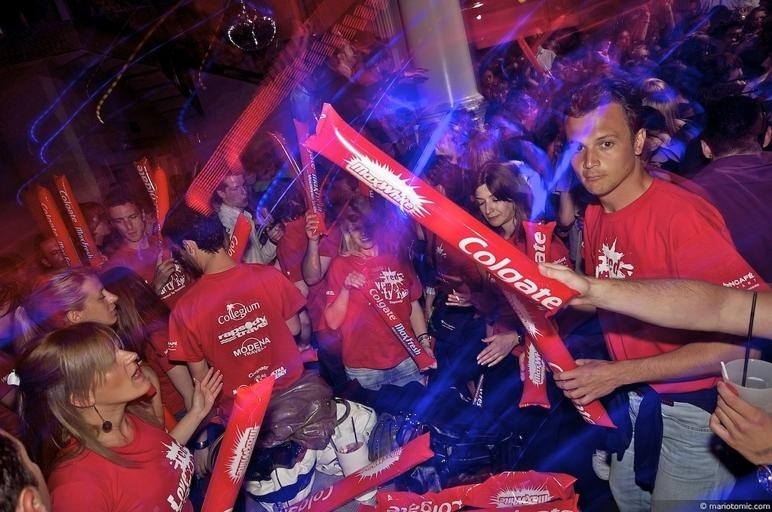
[0,0,772,510]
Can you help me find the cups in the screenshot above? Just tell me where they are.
[721,359,772,417]
[333,431,378,502]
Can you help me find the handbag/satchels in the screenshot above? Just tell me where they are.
[368,407,503,494]
[257,368,351,451]
